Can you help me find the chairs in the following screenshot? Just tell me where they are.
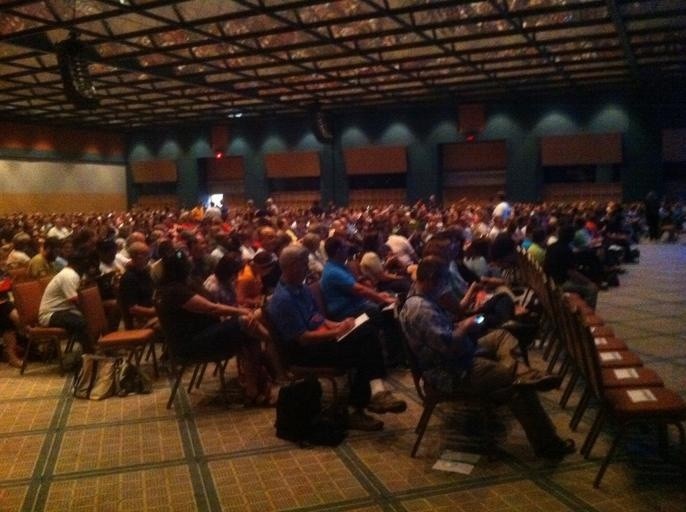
[11,282,65,376]
[39,276,53,288]
[155,299,235,410]
[76,284,159,398]
[262,294,350,427]
[401,333,505,462]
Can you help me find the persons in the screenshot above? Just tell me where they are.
[0,189,686,459]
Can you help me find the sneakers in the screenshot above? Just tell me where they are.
[541,438,575,457]
[514,370,563,394]
[368,389,407,414]
[349,410,384,431]
[4,357,25,367]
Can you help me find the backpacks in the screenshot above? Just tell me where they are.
[273,378,324,448]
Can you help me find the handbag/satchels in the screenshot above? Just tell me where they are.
[69,353,117,399]
[113,353,152,396]
